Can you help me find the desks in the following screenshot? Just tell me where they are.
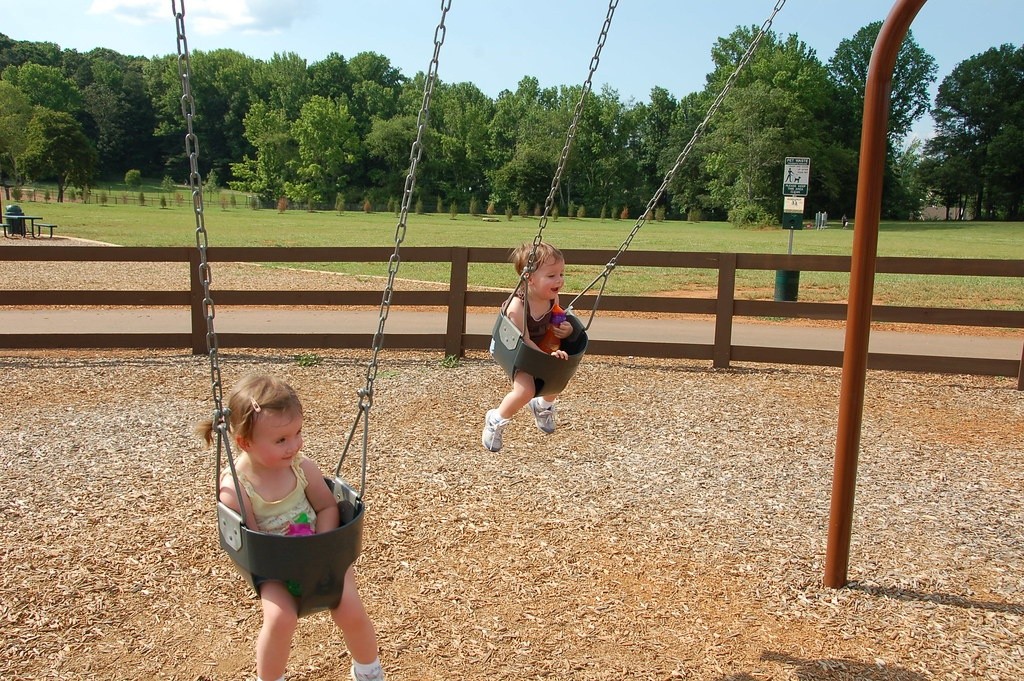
[2,215,53,239]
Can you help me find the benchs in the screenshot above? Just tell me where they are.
[35,223,58,227]
[0,224,10,227]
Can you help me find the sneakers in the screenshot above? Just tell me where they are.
[528,396,556,433]
[351,665,383,681]
[482,409,511,452]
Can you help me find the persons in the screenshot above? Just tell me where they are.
[842,214,847,229]
[481,242,573,452]
[909,211,914,221]
[199,375,385,681]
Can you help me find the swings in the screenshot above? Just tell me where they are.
[487,0,786,397]
[170,0,455,618]
